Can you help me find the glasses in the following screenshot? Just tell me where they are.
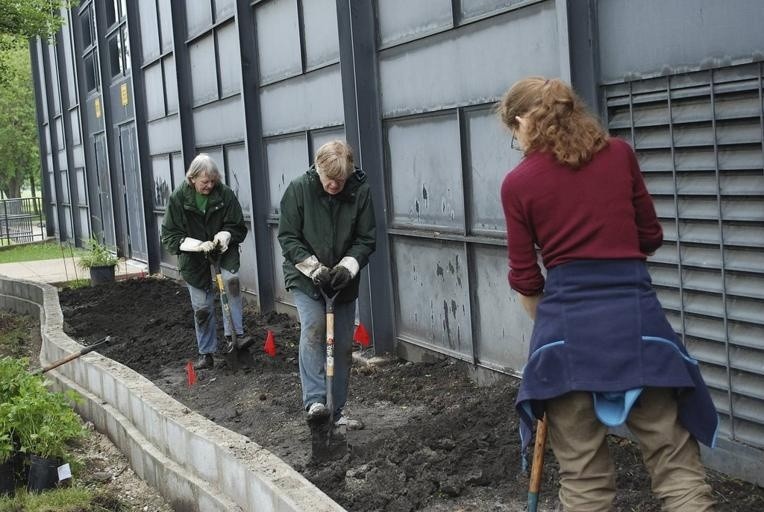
[510,127,522,151]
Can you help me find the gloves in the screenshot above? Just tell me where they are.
[294,255,360,290]
[178,231,232,253]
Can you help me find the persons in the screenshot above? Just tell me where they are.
[277,139,376,430]
[160,153,256,370]
[489,76,720,512]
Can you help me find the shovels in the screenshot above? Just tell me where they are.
[312,282,348,462]
[207,249,256,371]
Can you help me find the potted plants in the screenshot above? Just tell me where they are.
[0,357,92,500]
[72,231,120,288]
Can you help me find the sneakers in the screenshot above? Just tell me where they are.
[333,415,364,430]
[195,355,214,369]
[227,336,256,354]
[306,402,331,421]
